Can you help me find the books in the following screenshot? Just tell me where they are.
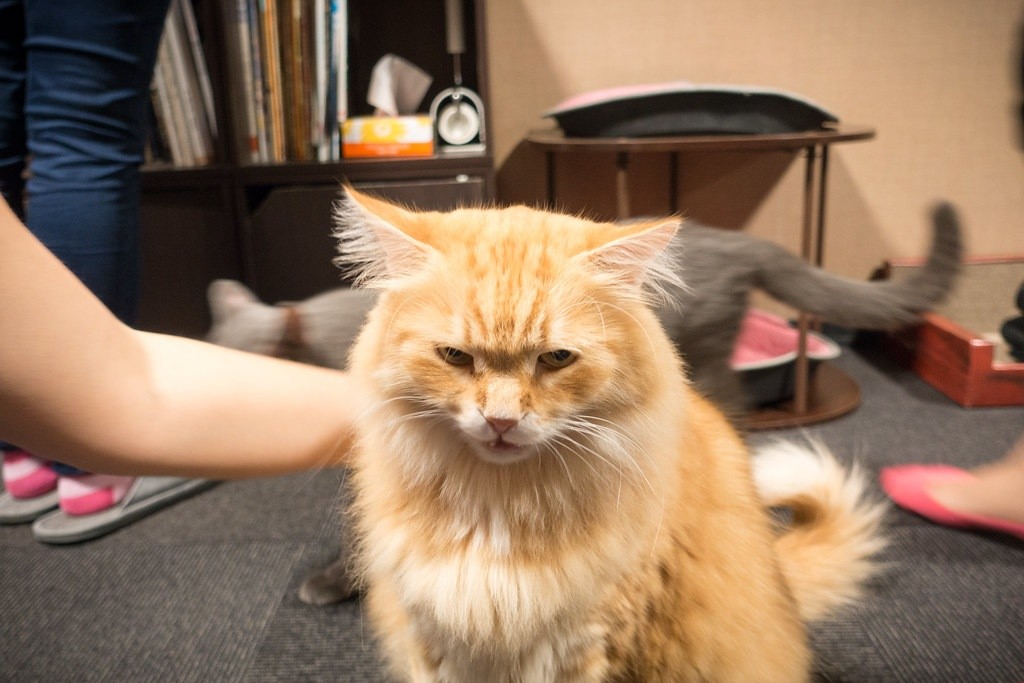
[142,0,347,166]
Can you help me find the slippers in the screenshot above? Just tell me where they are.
[0,489,62,526]
[33,465,219,543]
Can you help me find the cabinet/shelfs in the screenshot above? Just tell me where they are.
[130,0,498,341]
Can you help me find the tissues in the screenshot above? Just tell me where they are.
[340,55,435,159]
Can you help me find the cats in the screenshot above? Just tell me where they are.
[330,181,892,683]
[202,200,963,605]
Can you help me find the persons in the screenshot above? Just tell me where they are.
[0,0,218,542]
[0,196,358,480]
[882,16,1024,536]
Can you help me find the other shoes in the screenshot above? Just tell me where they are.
[881,462,1024,537]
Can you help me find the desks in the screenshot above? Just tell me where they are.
[524,126,878,433]
